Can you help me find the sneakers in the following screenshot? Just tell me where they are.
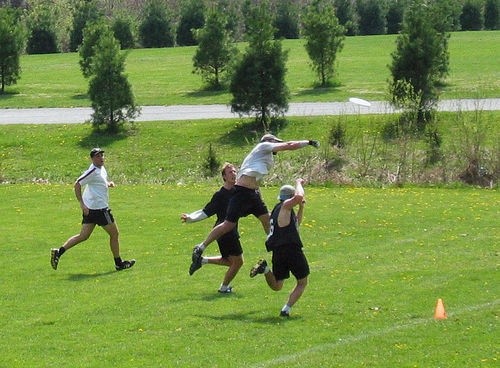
[281,311,289,316]
[51,249,59,270]
[250,259,267,278]
[116,260,136,270]
[193,244,203,262]
[218,287,233,294]
[189,257,203,275]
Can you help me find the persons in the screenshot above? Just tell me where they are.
[249,178,310,317]
[51,148,136,270]
[181,163,243,293]
[192,134,321,264]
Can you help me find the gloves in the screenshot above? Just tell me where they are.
[309,140,320,146]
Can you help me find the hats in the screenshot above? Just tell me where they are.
[90,148,104,156]
[278,185,296,200]
[261,134,283,142]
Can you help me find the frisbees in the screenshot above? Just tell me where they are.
[349,97,372,106]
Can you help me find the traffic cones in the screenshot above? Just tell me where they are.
[433,297,448,319]
